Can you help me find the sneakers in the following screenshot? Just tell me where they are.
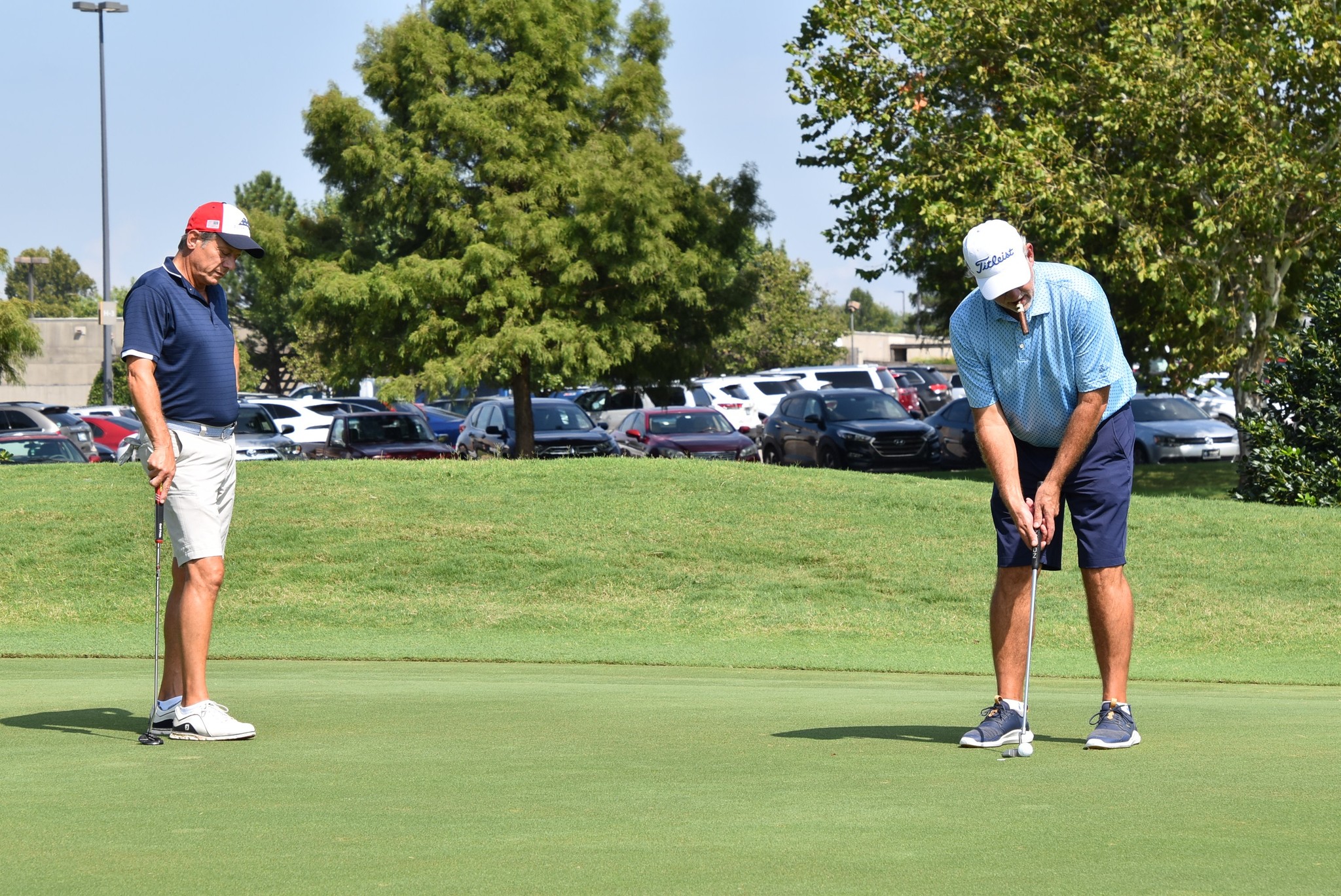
[147,700,182,734]
[960,695,1034,747]
[169,701,256,741]
[1083,698,1141,748]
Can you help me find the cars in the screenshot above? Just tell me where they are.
[1125,394,1237,467]
[923,391,986,465]
[655,363,951,433]
[0,400,449,464]
[425,395,753,465]
[760,390,947,479]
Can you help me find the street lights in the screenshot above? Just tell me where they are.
[895,290,906,330]
[847,300,859,365]
[75,0,126,403]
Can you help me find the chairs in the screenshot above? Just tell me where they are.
[677,418,697,432]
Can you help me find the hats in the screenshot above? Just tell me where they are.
[185,201,265,259]
[962,219,1031,300]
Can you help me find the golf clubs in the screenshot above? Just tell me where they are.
[1001,478,1045,758]
[137,481,166,745]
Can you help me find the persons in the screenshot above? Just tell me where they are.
[120,202,265,741]
[949,219,1142,749]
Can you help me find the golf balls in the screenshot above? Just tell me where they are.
[1017,742,1033,757]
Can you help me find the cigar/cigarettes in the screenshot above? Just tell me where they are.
[1015,300,1029,335]
